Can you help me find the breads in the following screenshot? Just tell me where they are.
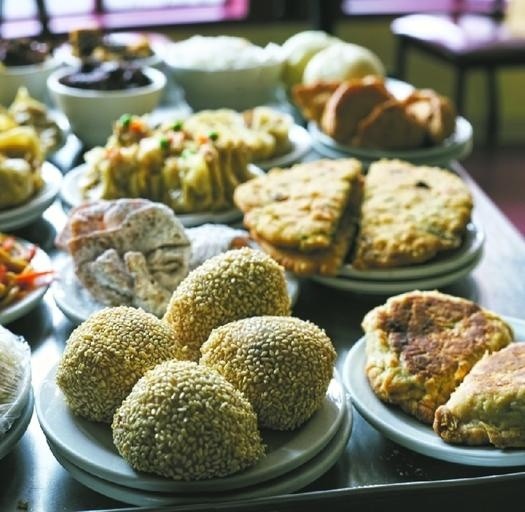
[290,75,455,153]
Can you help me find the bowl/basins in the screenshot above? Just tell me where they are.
[1,58,60,103]
[163,40,289,107]
[48,68,167,144]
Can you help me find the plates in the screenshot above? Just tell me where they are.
[61,161,267,225]
[344,318,525,468]
[39,357,352,508]
[0,237,50,321]
[307,110,475,169]
[311,215,487,291]
[2,325,35,460]
[53,228,298,324]
[0,159,65,230]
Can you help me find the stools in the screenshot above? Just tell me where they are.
[391,9,524,162]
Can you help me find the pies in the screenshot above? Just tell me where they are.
[360,291,514,425]
[234,160,473,275]
[432,339,525,448]
